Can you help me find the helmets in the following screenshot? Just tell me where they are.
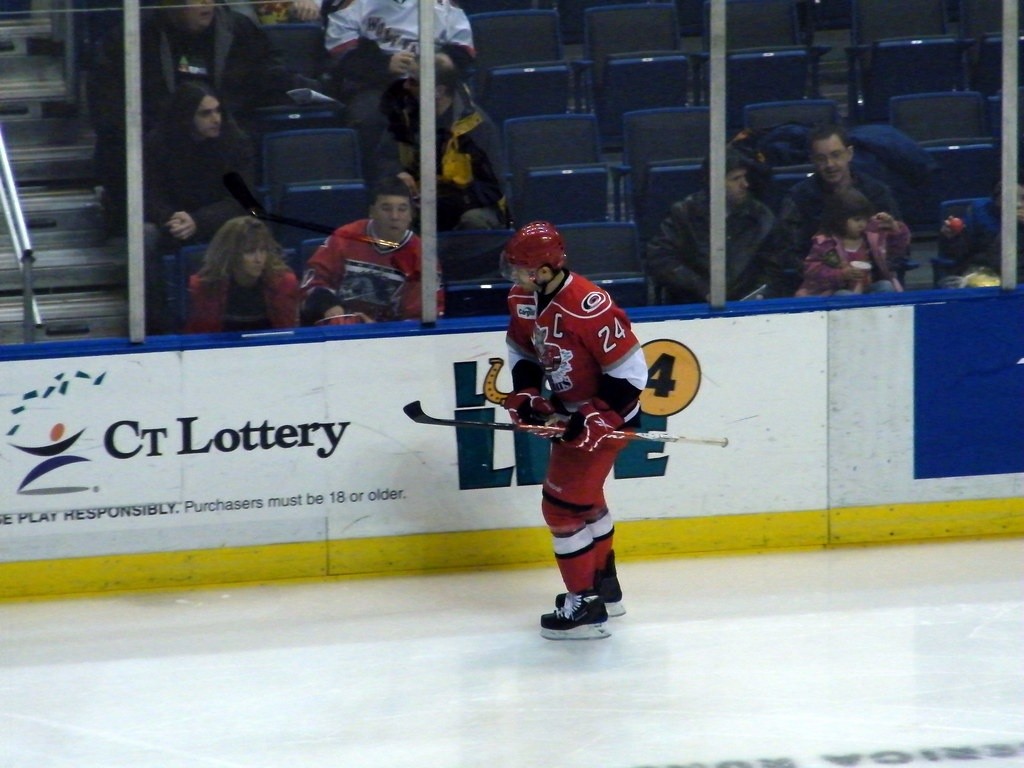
[499,221,565,286]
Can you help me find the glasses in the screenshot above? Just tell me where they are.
[808,148,847,165]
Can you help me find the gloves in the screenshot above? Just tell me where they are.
[509,388,557,439]
[559,396,625,452]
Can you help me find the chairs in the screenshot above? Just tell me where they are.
[171,0,1023,331]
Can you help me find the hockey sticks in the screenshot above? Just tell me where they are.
[400,398,729,449]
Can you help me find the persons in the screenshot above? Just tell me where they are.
[643,127,911,304]
[188,216,299,334]
[300,177,443,325]
[386,43,513,229]
[506,222,647,641]
[936,180,1024,285]
[136,0,478,143]
[146,80,250,246]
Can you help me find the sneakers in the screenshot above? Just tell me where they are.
[539,570,612,639]
[555,550,626,617]
